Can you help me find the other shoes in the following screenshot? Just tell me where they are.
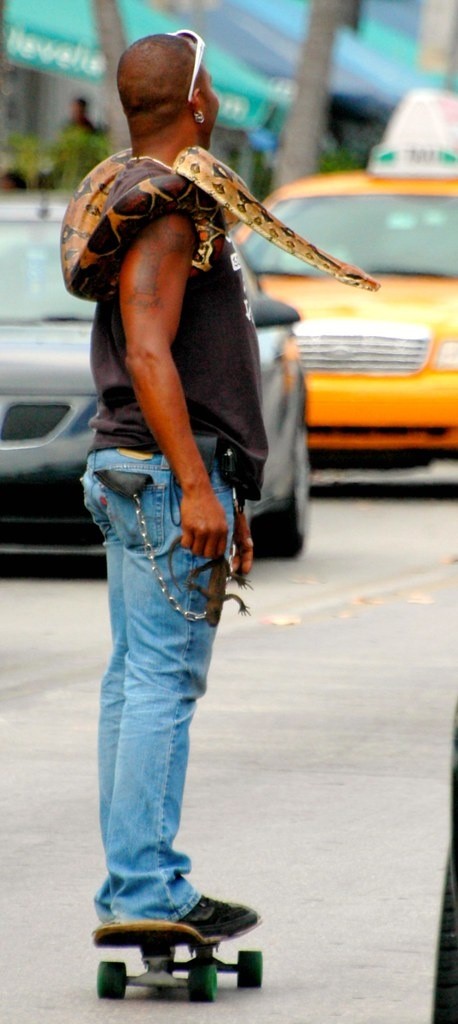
[176,894,262,944]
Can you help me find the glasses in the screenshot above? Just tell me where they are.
[168,29,206,103]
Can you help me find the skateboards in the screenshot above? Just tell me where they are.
[93,921,264,1001]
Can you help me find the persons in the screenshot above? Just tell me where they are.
[70,96,98,133]
[79,30,270,940]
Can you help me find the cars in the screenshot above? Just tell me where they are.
[0,182,314,574]
[232,140,458,498]
